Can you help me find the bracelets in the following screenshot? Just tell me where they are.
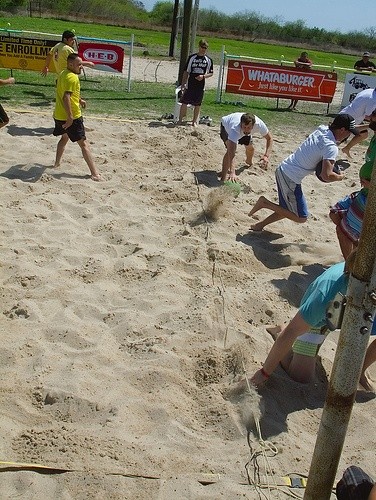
[370,117,372,121]
[261,368,269,377]
[203,75,204,78]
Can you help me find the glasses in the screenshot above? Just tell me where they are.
[200,45,207,49]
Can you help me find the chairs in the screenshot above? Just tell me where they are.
[174,84,192,122]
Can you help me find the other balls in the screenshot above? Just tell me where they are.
[224,180,242,197]
[315,159,341,182]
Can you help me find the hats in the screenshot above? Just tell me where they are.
[364,52,370,57]
[334,114,359,137]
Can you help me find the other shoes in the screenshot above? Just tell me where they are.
[288,107,291,112]
[166,113,173,119]
[200,116,205,123]
[204,116,212,124]
[161,113,167,118]
[293,106,298,111]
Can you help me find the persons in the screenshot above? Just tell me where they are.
[354,51,376,75]
[253,247,376,387]
[336,86,376,158]
[248,113,358,231]
[41,30,95,84]
[220,112,273,183]
[177,39,213,128]
[52,53,98,176]
[329,133,376,260]
[0,77,16,128]
[287,52,312,110]
